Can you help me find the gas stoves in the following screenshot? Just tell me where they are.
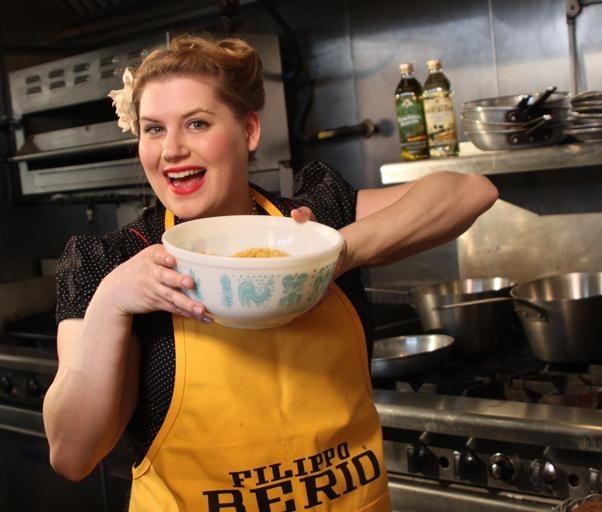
[0,303,59,406]
[367,325,601,511]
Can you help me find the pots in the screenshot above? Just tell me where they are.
[428,270,602,365]
[461,82,568,151]
[370,333,454,379]
[364,277,520,355]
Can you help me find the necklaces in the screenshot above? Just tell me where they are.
[176,188,257,225]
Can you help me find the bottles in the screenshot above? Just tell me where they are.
[395,64,432,161]
[422,59,460,160]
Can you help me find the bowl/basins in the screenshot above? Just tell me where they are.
[160,214,345,330]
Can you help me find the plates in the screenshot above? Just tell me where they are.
[561,88,602,143]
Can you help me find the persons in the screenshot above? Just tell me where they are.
[40,30,501,510]
